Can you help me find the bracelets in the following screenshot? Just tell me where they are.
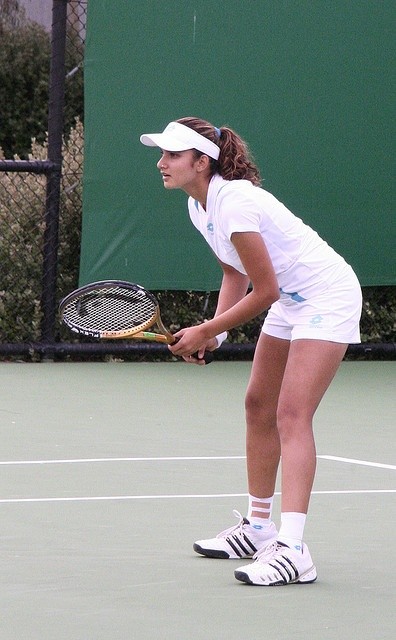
[214,330,228,349]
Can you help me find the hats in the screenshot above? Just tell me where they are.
[139,121,221,161]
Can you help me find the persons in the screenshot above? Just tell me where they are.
[139,117,364,586]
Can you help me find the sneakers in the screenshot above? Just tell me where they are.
[192,518,279,559]
[234,539,318,585]
